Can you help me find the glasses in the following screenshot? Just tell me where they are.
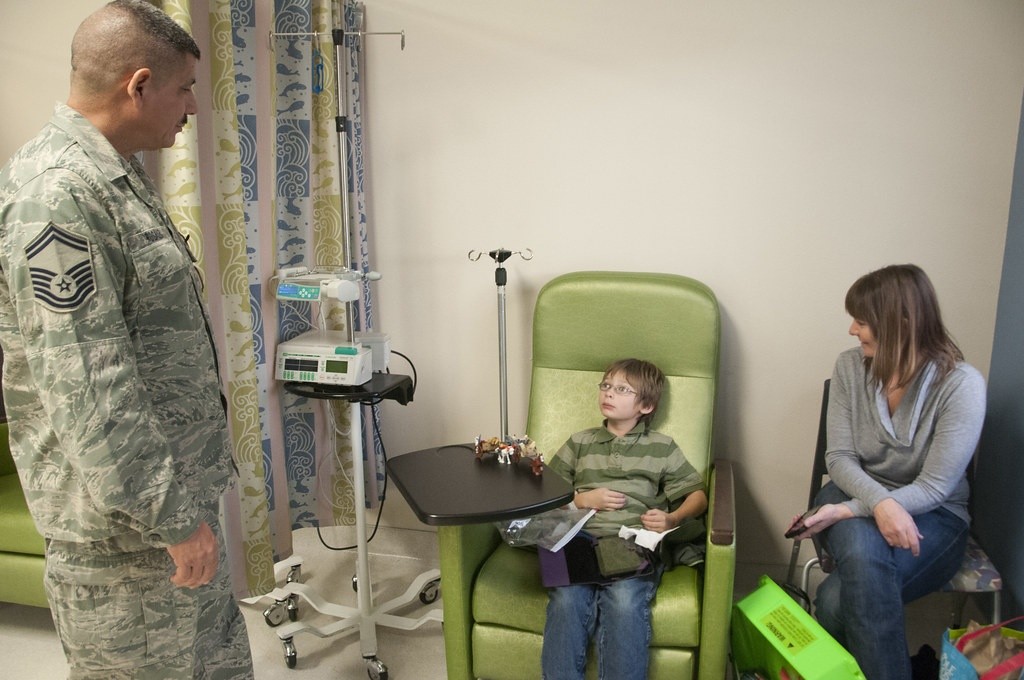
[598,383,638,396]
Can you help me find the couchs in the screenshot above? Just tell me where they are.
[0,422,49,608]
[441,270,738,680]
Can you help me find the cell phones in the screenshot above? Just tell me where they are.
[784,504,823,539]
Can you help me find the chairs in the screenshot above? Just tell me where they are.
[786,377,1003,627]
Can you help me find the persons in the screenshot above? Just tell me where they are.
[1,0,253,680]
[788,262,987,680]
[540,359,709,680]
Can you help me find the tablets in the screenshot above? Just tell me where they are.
[593,532,655,580]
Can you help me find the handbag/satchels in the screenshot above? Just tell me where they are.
[938,616,1024,680]
